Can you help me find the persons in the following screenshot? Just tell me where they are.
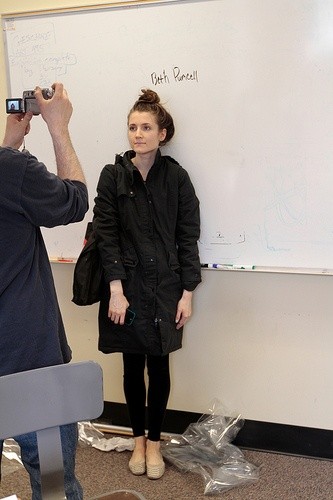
[10,103,15,111]
[93,89,204,479]
[0,81,89,500]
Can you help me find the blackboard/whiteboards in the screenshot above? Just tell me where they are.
[1,0,333,276]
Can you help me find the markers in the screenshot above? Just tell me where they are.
[201,264,254,270]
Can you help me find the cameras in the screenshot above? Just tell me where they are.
[6,87,55,116]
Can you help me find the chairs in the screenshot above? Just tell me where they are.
[0,360,146,500]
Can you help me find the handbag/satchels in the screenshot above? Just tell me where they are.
[70,221,103,308]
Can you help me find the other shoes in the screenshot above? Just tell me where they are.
[146,441,166,480]
[129,437,147,475]
